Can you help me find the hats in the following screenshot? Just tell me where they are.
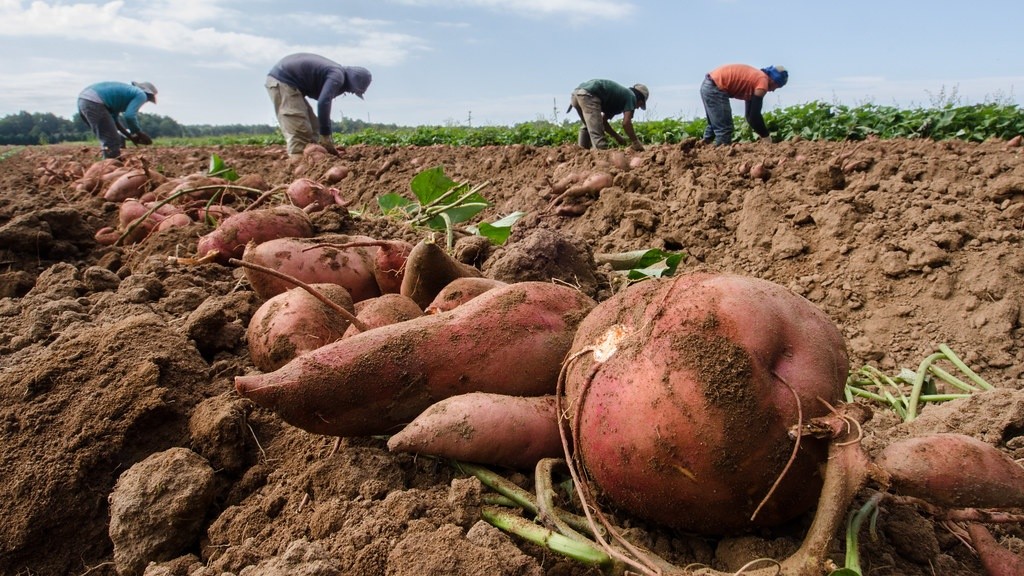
[761,65,788,87]
[132,81,158,104]
[347,66,372,100]
[630,84,649,110]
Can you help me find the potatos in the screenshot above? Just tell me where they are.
[26,146,1024,576]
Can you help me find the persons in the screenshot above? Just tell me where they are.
[571,79,649,152]
[77,81,159,162]
[700,64,789,146]
[264,53,372,158]
